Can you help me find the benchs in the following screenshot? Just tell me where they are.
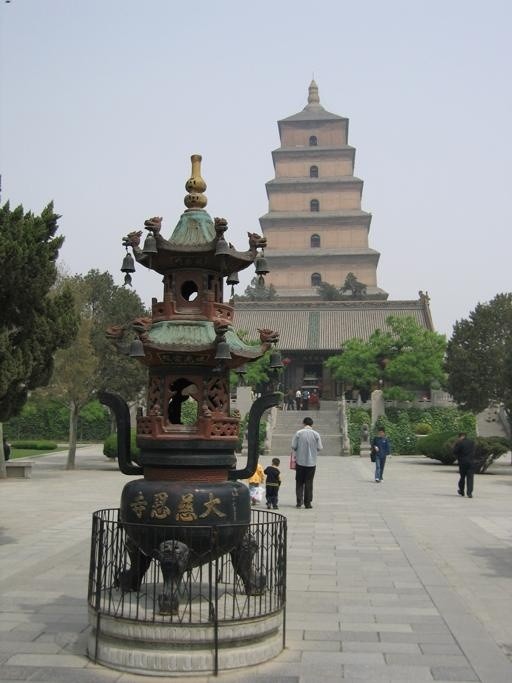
[5,461,32,479]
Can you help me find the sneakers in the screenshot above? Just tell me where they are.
[297,503,312,508]
[458,490,472,498]
[267,504,278,509]
[375,478,384,483]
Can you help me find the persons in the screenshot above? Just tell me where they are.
[247,457,281,509]
[453,432,477,498]
[284,388,308,410]
[291,417,323,509]
[371,426,390,482]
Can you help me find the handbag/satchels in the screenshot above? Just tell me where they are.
[289,455,296,469]
[371,453,375,462]
